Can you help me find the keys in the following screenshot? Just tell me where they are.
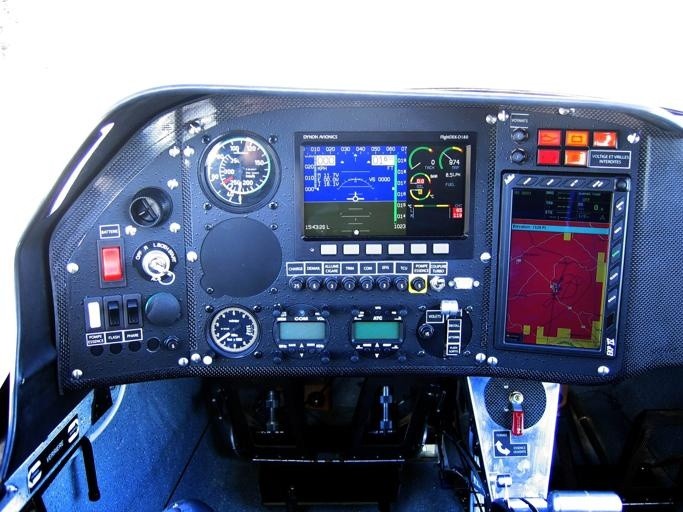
[150,262,173,279]
